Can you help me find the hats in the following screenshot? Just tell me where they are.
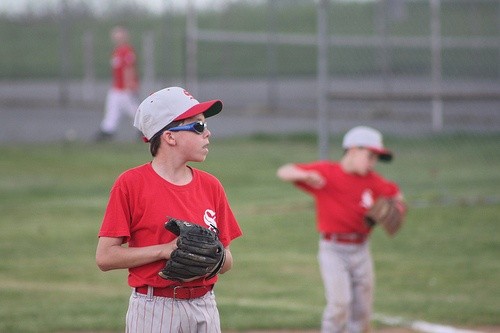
[343,125,396,163]
[132,86,222,143]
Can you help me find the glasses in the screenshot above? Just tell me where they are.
[165,121,212,136]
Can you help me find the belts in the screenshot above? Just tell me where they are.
[324,228,369,241]
[134,284,213,300]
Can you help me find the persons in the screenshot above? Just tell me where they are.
[95,85,244,333]
[275,125,407,332]
[87,25,146,143]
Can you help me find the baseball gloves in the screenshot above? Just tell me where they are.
[364,197,408,235]
[158,215,226,283]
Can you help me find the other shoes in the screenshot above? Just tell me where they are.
[89,132,111,142]
